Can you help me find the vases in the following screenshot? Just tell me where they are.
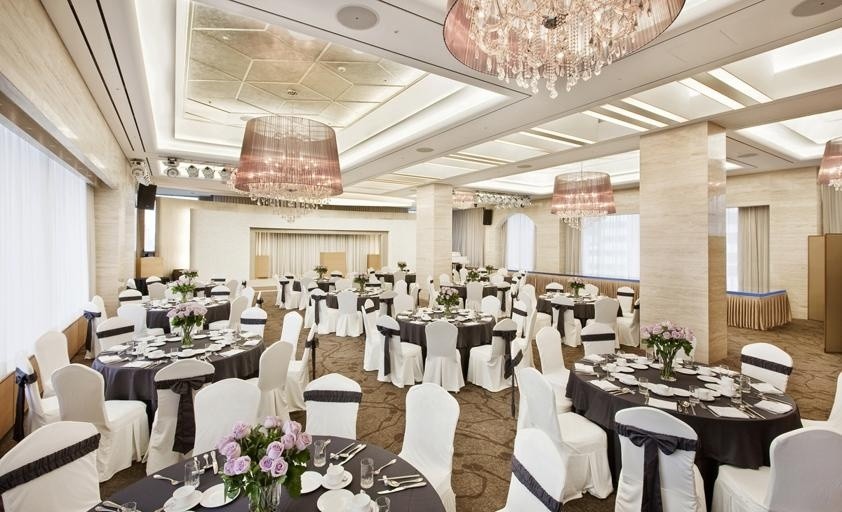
[248,481,281,511]
[658,349,678,381]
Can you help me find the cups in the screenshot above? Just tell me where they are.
[313,439,327,468]
[326,463,345,484]
[116,502,137,512]
[355,491,371,512]
[608,344,752,403]
[171,485,195,510]
[411,304,480,324]
[183,460,201,490]
[124,321,243,363]
[359,457,376,490]
[374,496,391,512]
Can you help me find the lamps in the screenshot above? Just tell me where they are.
[129,159,151,187]
[232,89,343,225]
[549,161,617,233]
[441,0,683,102]
[451,191,474,211]
[813,135,841,195]
[162,156,234,184]
[472,190,535,211]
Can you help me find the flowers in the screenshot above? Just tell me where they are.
[219,416,312,511]
[641,322,694,378]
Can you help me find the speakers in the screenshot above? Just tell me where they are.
[137,182,157,211]
[483,209,492,225]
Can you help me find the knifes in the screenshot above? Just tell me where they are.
[94,500,142,512]
[338,445,366,465]
[764,395,791,405]
[737,404,766,420]
[678,399,682,412]
[376,481,427,494]
[209,450,219,475]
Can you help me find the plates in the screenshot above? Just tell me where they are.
[163,490,204,512]
[145,296,216,310]
[545,299,551,301]
[199,483,241,509]
[299,470,322,495]
[370,500,380,512]
[317,490,353,512]
[321,471,352,490]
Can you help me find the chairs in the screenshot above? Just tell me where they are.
[193,378,261,455]
[274,259,423,338]
[396,383,461,512]
[288,324,316,411]
[614,406,706,512]
[244,341,292,421]
[711,426,842,512]
[146,358,215,475]
[740,342,792,392]
[802,371,842,429]
[82,267,268,442]
[303,373,361,440]
[34,331,71,399]
[16,353,60,439]
[51,363,150,481]
[279,311,303,361]
[364,250,641,413]
[519,367,614,498]
[496,427,567,512]
[0,421,102,512]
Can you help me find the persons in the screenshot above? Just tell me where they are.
[452,262,467,284]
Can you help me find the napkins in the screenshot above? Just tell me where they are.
[754,400,792,415]
[709,406,748,418]
[712,367,739,377]
[648,398,677,411]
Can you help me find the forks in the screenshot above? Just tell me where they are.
[153,474,184,486]
[698,402,719,417]
[371,458,397,474]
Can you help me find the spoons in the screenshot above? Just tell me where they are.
[192,453,213,476]
[339,444,361,458]
[682,399,689,414]
[386,478,424,486]
